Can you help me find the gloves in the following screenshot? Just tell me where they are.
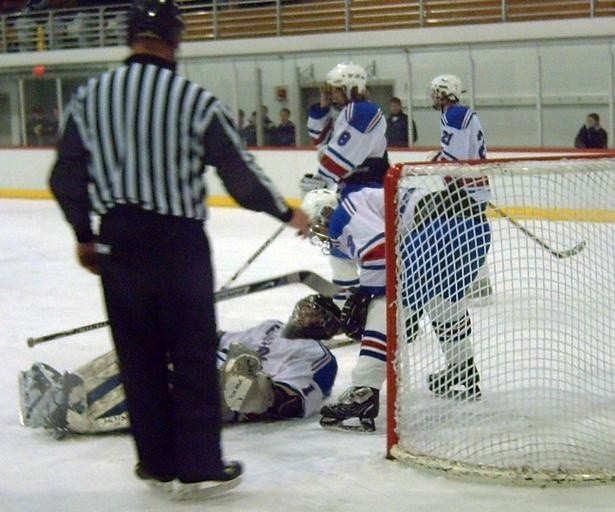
[298,175,328,193]
[222,370,276,415]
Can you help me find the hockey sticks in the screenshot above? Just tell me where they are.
[27,272,346,347]
[486,201,586,259]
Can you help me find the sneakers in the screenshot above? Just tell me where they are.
[425,356,481,386]
[319,385,381,420]
[131,454,245,486]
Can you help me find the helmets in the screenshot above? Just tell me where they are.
[296,187,340,244]
[281,292,342,341]
[323,60,369,104]
[126,0,186,51]
[429,73,464,110]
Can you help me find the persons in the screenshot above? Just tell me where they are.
[297,62,390,345]
[26,103,49,146]
[17,294,342,435]
[240,106,296,146]
[383,97,417,147]
[574,113,608,149]
[301,181,491,419]
[429,73,493,299]
[49,106,60,138]
[48,0,312,500]
